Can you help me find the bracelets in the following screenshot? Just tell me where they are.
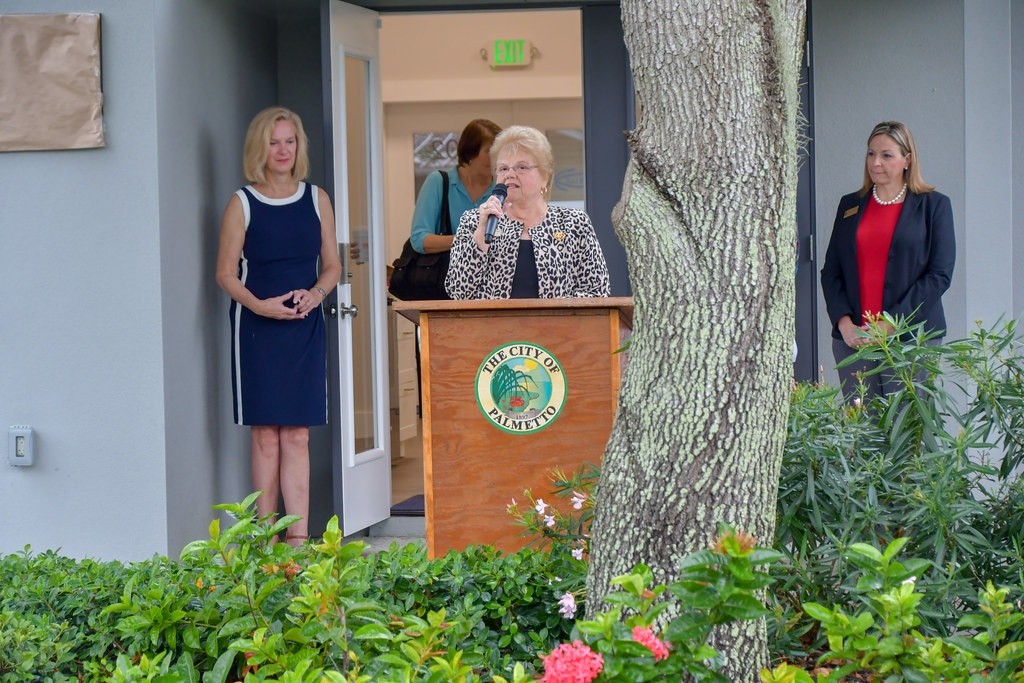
[312,287,326,298]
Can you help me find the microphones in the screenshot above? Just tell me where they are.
[484,182,509,244]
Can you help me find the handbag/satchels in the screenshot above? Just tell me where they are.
[389,170,454,299]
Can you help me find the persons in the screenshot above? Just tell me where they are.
[409,119,503,255]
[442,126,611,298]
[214,108,343,547]
[820,122,956,425]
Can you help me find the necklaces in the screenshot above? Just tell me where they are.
[873,183,907,206]
[507,202,547,232]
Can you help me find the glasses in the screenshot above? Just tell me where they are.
[495,163,541,176]
